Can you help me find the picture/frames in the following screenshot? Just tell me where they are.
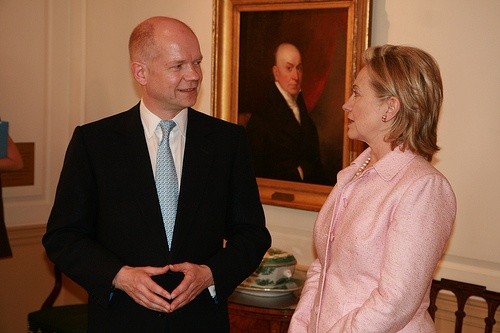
[210,0,374,208]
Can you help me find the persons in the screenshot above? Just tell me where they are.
[41,16,272,333]
[0,119,24,259]
[287,44,457,333]
[253,43,324,184]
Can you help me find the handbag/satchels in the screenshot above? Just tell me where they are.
[26,261,91,333]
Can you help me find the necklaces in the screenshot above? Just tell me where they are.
[352,157,371,178]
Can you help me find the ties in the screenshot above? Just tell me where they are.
[155,119,179,253]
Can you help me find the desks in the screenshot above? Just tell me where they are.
[228,268,306,333]
